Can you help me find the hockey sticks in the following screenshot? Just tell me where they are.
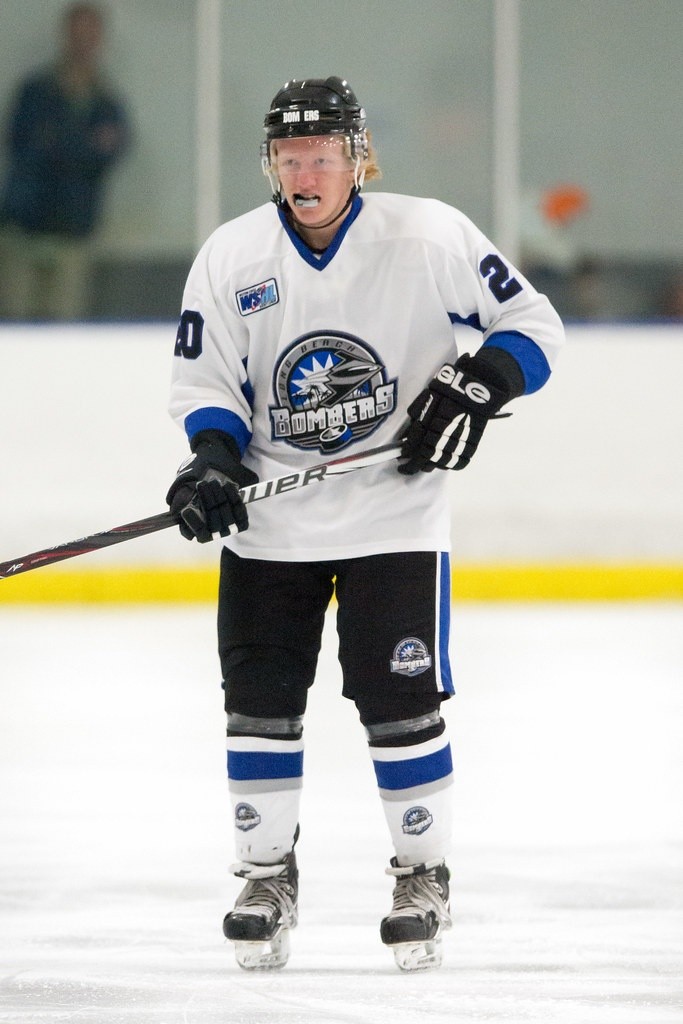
[0,412,516,581]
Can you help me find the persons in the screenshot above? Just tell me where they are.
[0,0,143,329]
[166,76,565,978]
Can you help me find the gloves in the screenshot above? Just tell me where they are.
[166,428,260,544]
[397,351,510,477]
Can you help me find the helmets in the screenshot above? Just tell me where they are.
[259,76,370,164]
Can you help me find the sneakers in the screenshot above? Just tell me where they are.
[222,824,300,972]
[379,856,454,976]
